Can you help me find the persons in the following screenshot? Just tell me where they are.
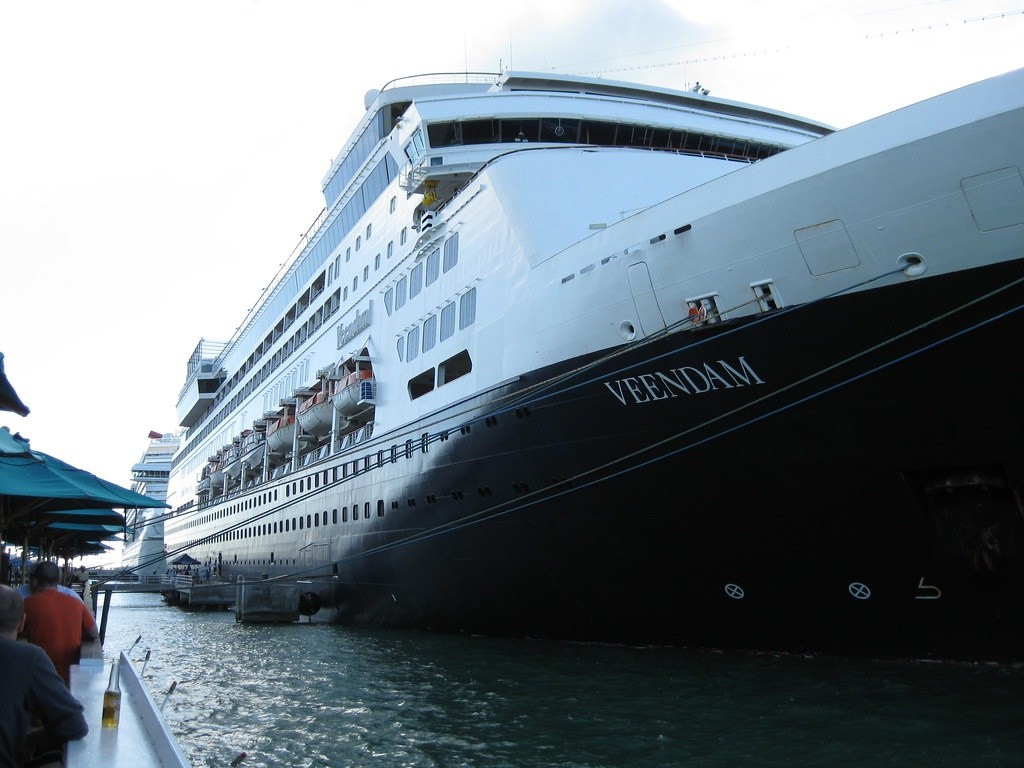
[166,559,217,586]
[0,561,99,768]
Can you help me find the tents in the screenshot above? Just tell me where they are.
[168,553,201,574]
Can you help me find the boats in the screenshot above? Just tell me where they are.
[240,431,266,469]
[297,391,356,443]
[222,447,242,480]
[266,415,318,459]
[331,370,375,422]
[210,463,224,488]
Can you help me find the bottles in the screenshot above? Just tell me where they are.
[102,659,122,728]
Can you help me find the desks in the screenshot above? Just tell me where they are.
[66,664,163,768]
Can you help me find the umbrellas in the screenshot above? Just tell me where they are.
[0,426,172,584]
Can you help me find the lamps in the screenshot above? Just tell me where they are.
[421,180,438,207]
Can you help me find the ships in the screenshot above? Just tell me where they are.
[122,430,181,581]
[162,59,1024,664]
